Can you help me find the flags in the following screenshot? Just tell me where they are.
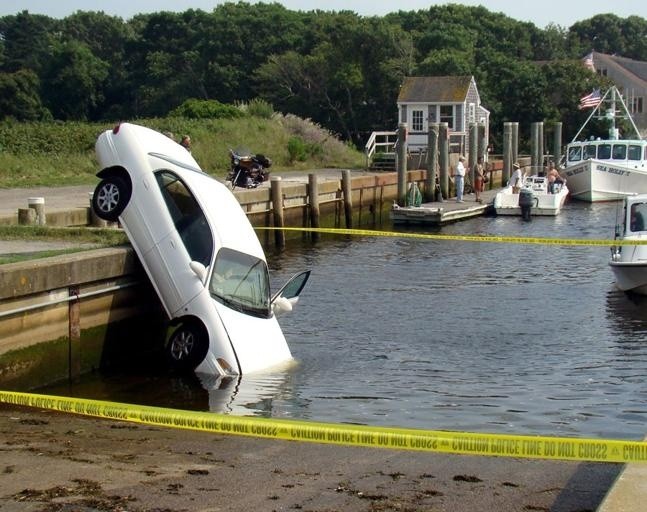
[577,89,601,111]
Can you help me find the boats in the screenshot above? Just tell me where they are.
[556,85,647,205]
[610,193,647,295]
[495,165,570,218]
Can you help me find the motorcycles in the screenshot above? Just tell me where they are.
[226,145,272,191]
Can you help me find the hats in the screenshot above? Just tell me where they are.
[512,162,520,168]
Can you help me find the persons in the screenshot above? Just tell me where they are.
[474,156,484,202]
[545,161,565,194]
[454,155,467,204]
[508,162,521,194]
[165,131,173,138]
[179,135,191,154]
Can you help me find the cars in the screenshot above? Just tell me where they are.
[92,121,311,390]
